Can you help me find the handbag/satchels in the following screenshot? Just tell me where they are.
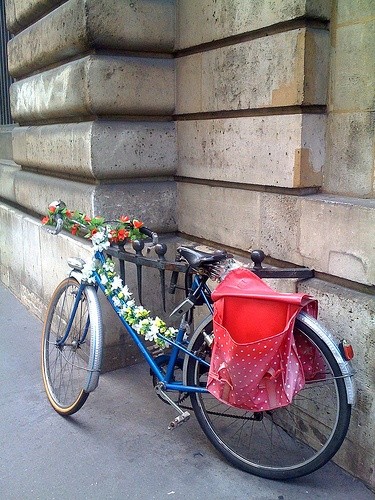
[206,267,325,412]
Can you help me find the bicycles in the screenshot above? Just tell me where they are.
[39,198,356,480]
